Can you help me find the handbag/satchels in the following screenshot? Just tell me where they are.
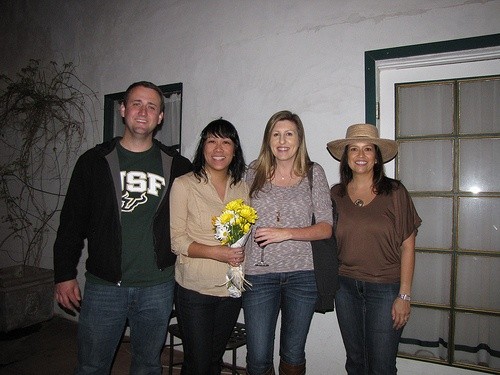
[306,160,339,313]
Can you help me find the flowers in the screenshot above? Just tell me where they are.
[210,198,260,299]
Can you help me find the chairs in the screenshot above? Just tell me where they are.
[167,307,248,375]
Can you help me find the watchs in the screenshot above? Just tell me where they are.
[399,294,410,301]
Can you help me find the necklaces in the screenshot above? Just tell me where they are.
[354,198,368,207]
[277,168,291,180]
[274,173,299,222]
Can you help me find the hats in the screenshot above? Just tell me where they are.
[326,123,399,165]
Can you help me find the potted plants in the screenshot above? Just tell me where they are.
[0,54,103,336]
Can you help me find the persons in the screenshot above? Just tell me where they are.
[52,80,195,375]
[245,110,333,375]
[326,124,422,375]
[169,119,251,375]
